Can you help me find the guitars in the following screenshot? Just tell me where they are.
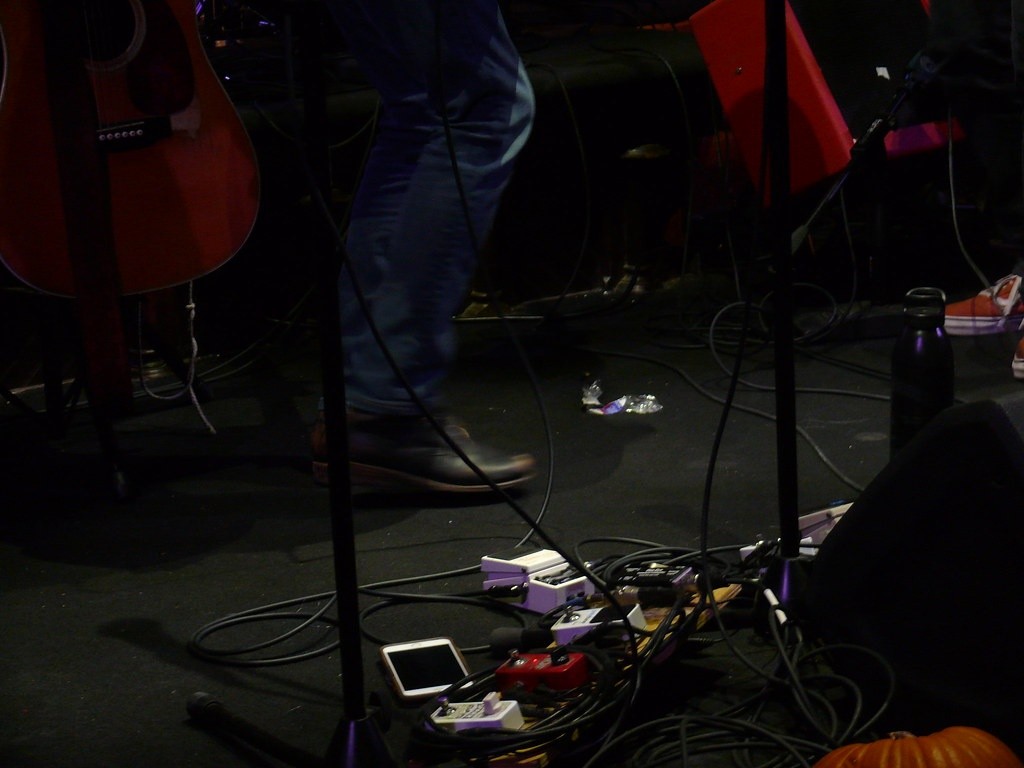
[0,1,261,302]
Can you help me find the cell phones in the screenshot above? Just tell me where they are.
[378,636,475,702]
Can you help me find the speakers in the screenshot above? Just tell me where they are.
[796,391,1024,765]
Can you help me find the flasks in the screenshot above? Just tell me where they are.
[888,286,959,460]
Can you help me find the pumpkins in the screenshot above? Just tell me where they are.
[816,725,1016,768]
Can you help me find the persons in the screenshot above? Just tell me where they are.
[309,0,538,493]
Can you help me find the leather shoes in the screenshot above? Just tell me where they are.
[310,409,541,493]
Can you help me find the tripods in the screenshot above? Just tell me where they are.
[683,0,814,635]
[187,0,394,768]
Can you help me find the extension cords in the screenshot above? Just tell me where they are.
[430,701,525,734]
